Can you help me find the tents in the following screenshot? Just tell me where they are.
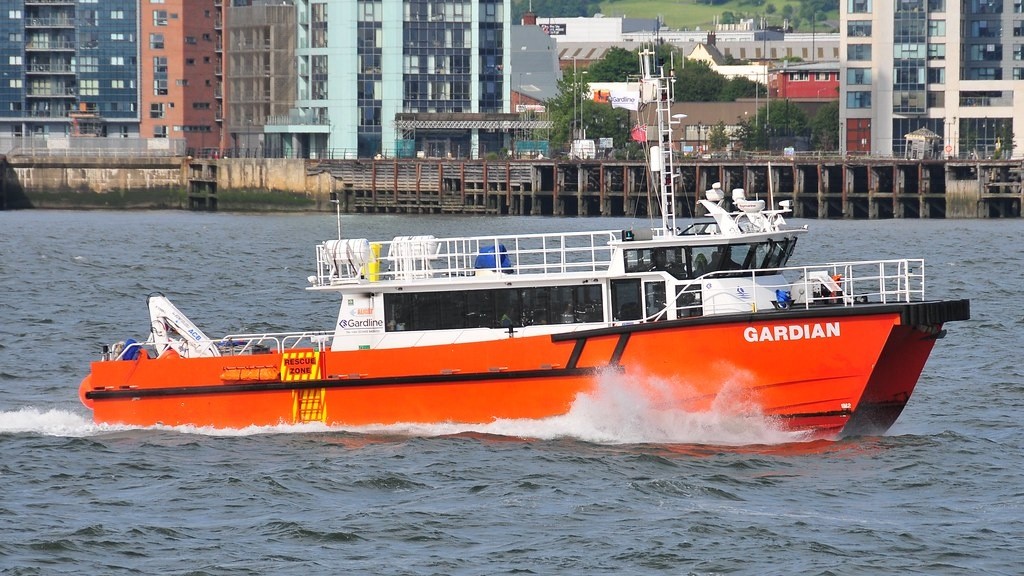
[904,124,941,159]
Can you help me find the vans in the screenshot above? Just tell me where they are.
[567,140,595,161]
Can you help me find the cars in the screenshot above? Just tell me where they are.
[690,152,702,158]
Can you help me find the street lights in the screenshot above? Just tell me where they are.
[580,71,588,139]
[744,111,758,151]
[518,72,532,109]
[703,127,707,153]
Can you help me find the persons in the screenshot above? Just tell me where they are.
[651,248,667,270]
[560,303,575,324]
[499,305,513,327]
[693,251,720,277]
[972,147,980,160]
[718,247,741,277]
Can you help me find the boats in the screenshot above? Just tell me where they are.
[77,49,971,440]
